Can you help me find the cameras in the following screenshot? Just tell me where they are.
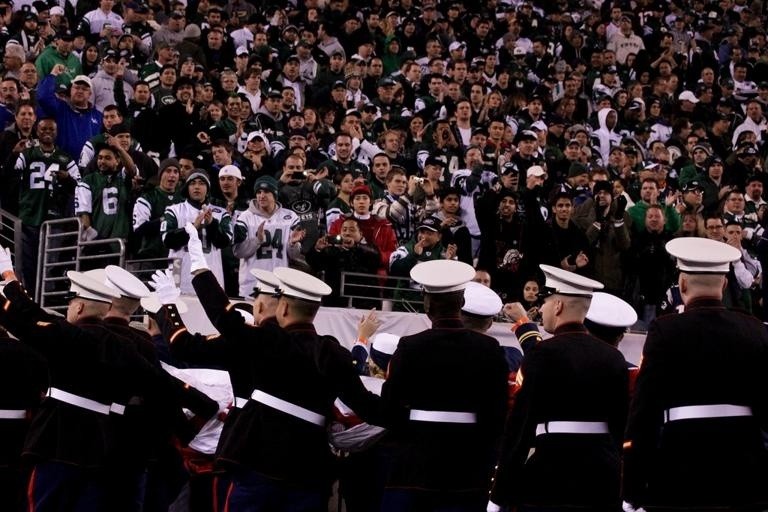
[414,177,424,184]
[328,236,343,244]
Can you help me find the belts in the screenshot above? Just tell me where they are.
[407,411,481,425]
[110,403,127,418]
[229,398,248,405]
[1,409,28,425]
[45,389,114,417]
[249,389,324,427]
[536,422,612,437]
[662,404,729,418]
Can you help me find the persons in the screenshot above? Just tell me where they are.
[309,218,384,311]
[215,0,233,141]
[317,131,370,179]
[582,289,639,374]
[233,131,272,175]
[132,158,186,265]
[687,155,737,210]
[233,176,306,302]
[372,2,435,166]
[626,1,686,178]
[532,0,570,193]
[0,98,40,172]
[697,214,753,306]
[372,168,437,242]
[40,62,106,164]
[325,187,398,266]
[86,45,136,112]
[718,190,767,234]
[470,270,512,323]
[438,2,470,186]
[633,204,679,309]
[264,154,334,231]
[532,194,594,274]
[125,80,156,121]
[0,270,218,512]
[334,305,402,502]
[234,1,271,129]
[721,221,757,274]
[271,2,315,151]
[628,237,768,510]
[386,216,464,312]
[0,0,34,65]
[209,142,253,182]
[669,210,711,238]
[0,78,30,128]
[84,0,150,43]
[573,182,630,282]
[571,1,624,182]
[13,117,81,242]
[731,130,767,174]
[160,77,213,161]
[470,2,530,168]
[0,244,190,512]
[113,56,153,152]
[508,278,544,325]
[419,188,472,266]
[97,124,160,194]
[33,27,83,87]
[16,63,40,97]
[383,258,510,512]
[486,264,627,506]
[74,137,139,270]
[625,178,682,232]
[184,220,284,512]
[34,1,83,25]
[454,282,543,374]
[78,105,121,172]
[731,2,767,98]
[154,1,215,76]
[209,165,247,220]
[732,100,767,151]
[670,179,709,217]
[174,154,203,183]
[139,288,188,369]
[148,265,387,512]
[688,0,730,153]
[318,0,370,129]
[161,170,234,295]
[472,170,531,274]
[741,176,768,214]
[79,40,99,78]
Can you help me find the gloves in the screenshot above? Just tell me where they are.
[593,196,608,221]
[183,219,210,272]
[148,268,181,304]
[0,245,14,274]
[615,194,627,227]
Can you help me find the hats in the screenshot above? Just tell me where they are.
[568,139,579,147]
[370,331,403,371]
[218,164,247,181]
[178,53,193,64]
[692,144,710,154]
[60,26,76,40]
[535,264,605,299]
[296,39,312,48]
[387,10,396,18]
[426,154,448,167]
[609,145,622,153]
[184,22,202,38]
[253,174,279,195]
[63,269,121,302]
[50,5,65,16]
[513,45,525,57]
[567,161,588,176]
[501,161,518,174]
[272,266,332,302]
[713,111,732,122]
[514,130,536,142]
[597,94,611,103]
[665,236,741,272]
[103,48,116,59]
[593,180,612,195]
[707,155,725,166]
[111,124,132,136]
[460,281,502,316]
[624,146,638,154]
[237,44,249,56]
[246,130,264,142]
[126,1,143,14]
[158,157,181,176]
[418,215,441,232]
[332,79,346,89]
[267,90,281,98]
[410,259,475,291]
[528,92,542,101]
[530,120,547,130]
[634,120,655,133]
[375,76,395,85]
[179,168,211,197]
[677,90,698,103]
[527,165,549,180]
[450,39,465,52]
[73,73,92,88]
[331,48,346,56]
[681,180,706,191]
[105,262,150,299]
[282,23,299,33]
[345,108,361,117]
[344,63,363,78]
[250,267,282,300]
[349,184,371,196]
[287,55,298,62]
[586,291,638,328]
[619,14,631,22]
[140,290,188,319]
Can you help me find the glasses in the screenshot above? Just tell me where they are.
[708,224,725,230]
[730,229,741,236]
[363,107,377,113]
[728,196,744,202]
[289,136,304,142]
[24,68,37,73]
[4,54,12,60]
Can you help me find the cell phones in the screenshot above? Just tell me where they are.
[292,172,306,179]
